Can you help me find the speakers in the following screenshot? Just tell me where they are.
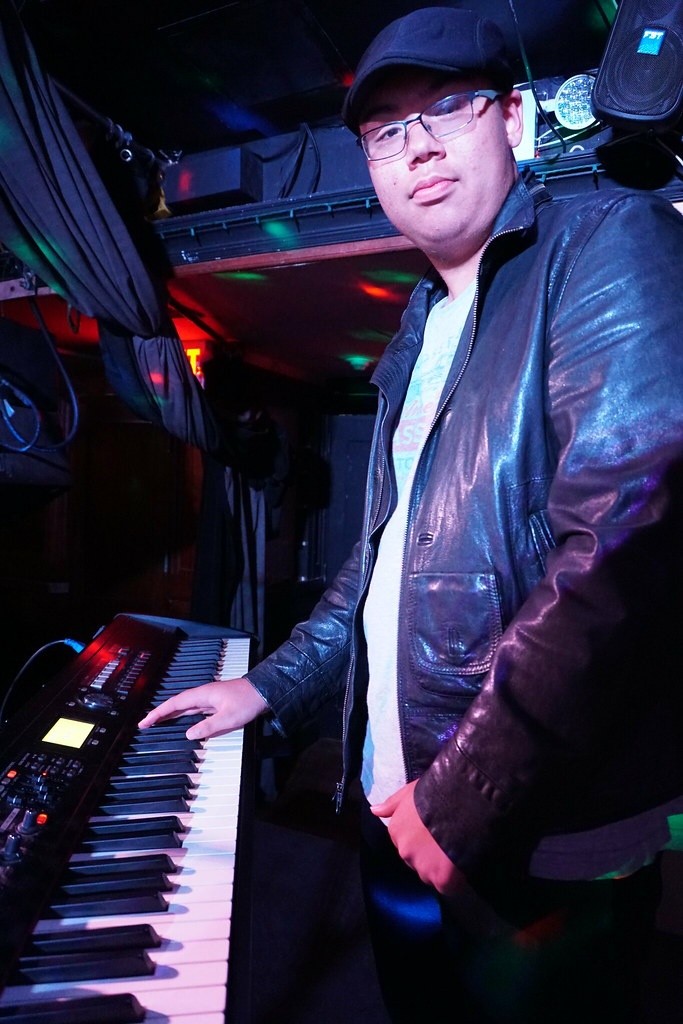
[590,0,683,127]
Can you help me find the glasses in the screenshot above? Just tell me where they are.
[356,89,503,160]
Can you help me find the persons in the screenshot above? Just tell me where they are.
[137,8,683,1024]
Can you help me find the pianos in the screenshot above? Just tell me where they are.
[0,611,260,1024]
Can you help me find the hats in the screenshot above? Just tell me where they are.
[341,7,513,136]
